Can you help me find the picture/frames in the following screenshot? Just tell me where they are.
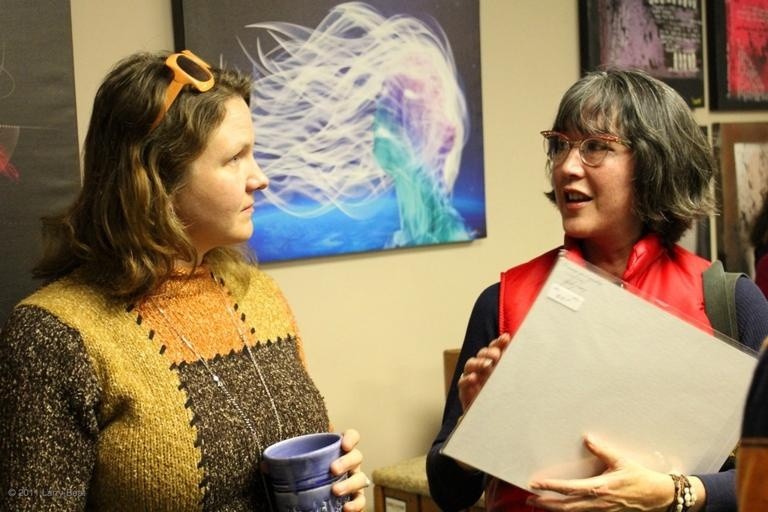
[707,1,767,112]
[675,125,712,265]
[575,1,703,112]
[712,121,768,301]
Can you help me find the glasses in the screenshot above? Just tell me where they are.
[539,129,622,169]
[145,50,215,136]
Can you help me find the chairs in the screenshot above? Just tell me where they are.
[369,348,487,512]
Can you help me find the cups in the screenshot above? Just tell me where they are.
[260,433,350,512]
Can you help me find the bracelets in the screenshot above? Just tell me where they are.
[668,473,696,512]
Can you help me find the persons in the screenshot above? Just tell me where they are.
[425,67,768,512]
[1,48,370,512]
